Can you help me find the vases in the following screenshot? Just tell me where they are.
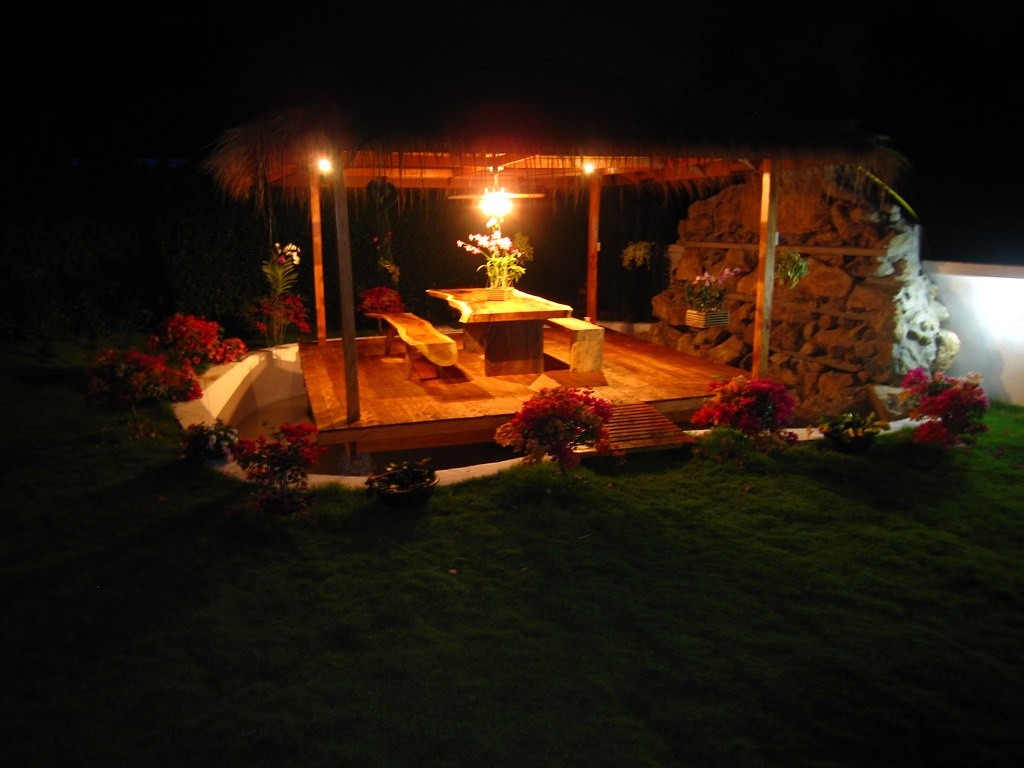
[484,287,514,301]
[205,455,227,467]
[685,310,731,328]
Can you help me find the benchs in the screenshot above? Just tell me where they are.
[545,317,605,374]
[365,312,458,380]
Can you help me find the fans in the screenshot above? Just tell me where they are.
[448,174,546,200]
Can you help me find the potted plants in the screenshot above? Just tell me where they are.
[807,411,891,452]
[364,455,438,507]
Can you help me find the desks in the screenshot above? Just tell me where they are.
[425,288,573,377]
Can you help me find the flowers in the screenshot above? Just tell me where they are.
[690,374,801,452]
[246,293,311,347]
[685,267,735,312]
[457,218,527,288]
[84,347,202,438]
[898,366,991,446]
[357,286,407,336]
[224,416,329,520]
[223,337,248,364]
[262,242,301,295]
[176,418,240,468]
[142,311,225,376]
[493,383,625,478]
